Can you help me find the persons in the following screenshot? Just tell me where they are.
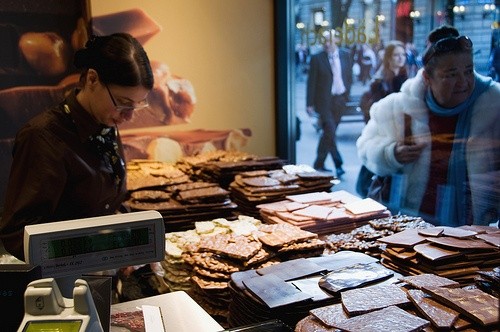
[0,31,154,277]
[360,39,409,125]
[490,28,500,78]
[424,25,459,50]
[357,24,500,227]
[294,43,313,84]
[404,42,419,78]
[304,27,354,177]
[349,43,385,86]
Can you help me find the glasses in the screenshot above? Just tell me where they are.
[106,85,149,113]
[423,35,473,64]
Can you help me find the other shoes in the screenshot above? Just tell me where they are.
[315,166,331,173]
[336,168,345,177]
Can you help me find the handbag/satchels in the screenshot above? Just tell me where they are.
[359,76,393,126]
[365,113,411,206]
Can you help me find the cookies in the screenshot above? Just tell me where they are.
[124,150,500,332]
